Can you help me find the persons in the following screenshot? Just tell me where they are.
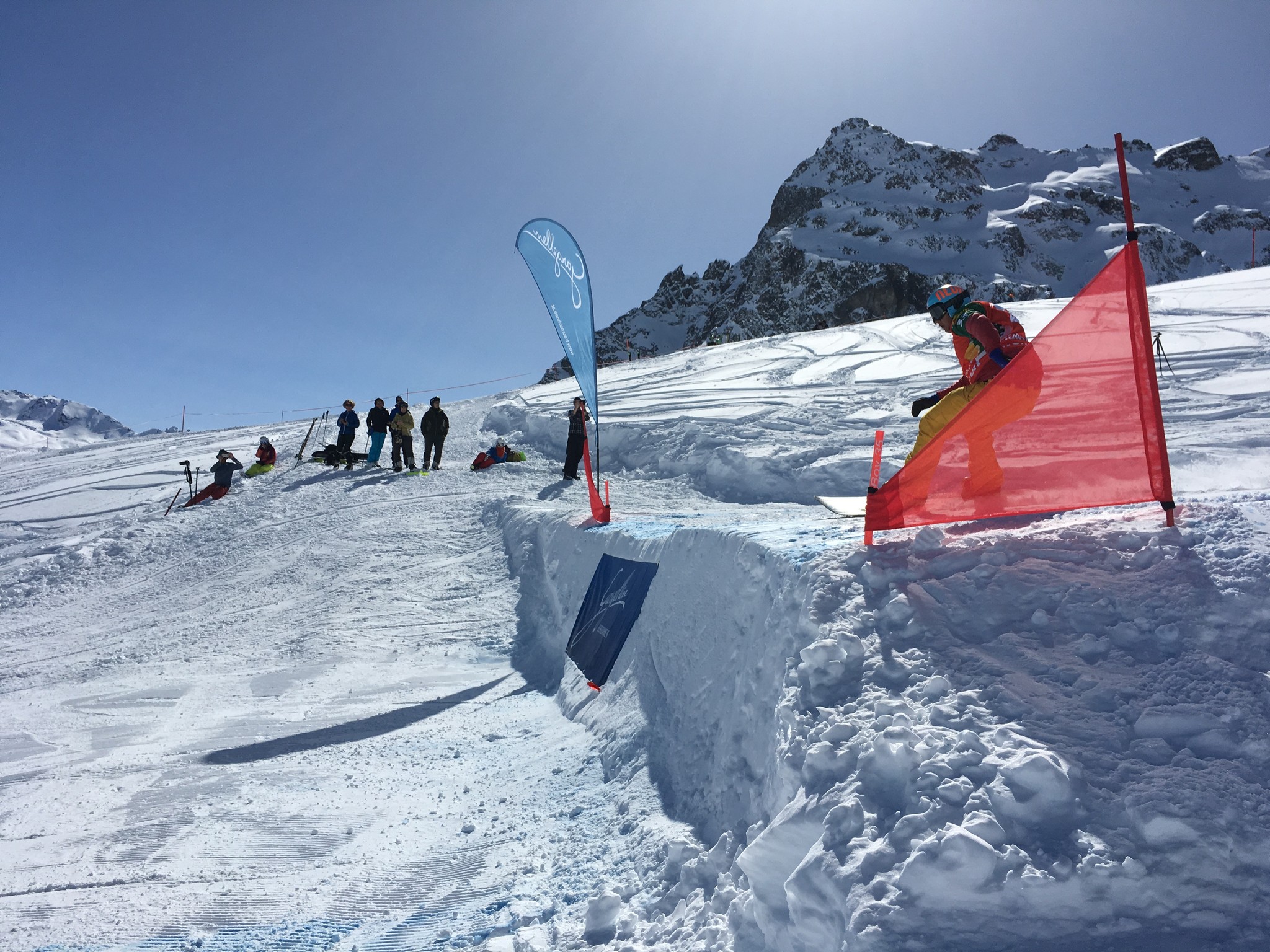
[333,399,360,470]
[238,436,277,478]
[388,395,411,468]
[899,283,1043,510]
[562,396,590,480]
[1007,294,1014,302]
[177,449,243,507]
[366,397,391,468]
[391,401,418,472]
[470,437,507,471]
[420,396,449,470]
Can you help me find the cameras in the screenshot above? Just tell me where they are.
[179,460,190,466]
[263,442,266,445]
[579,400,586,405]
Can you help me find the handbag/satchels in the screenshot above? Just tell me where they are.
[505,450,526,462]
[324,444,337,466]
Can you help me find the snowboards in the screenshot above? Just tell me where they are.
[813,495,867,516]
[312,451,369,463]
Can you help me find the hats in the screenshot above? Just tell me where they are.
[573,397,581,405]
[216,449,229,460]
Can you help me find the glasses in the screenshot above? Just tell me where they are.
[345,404,352,407]
[376,402,383,404]
[260,441,268,446]
[400,403,407,407]
[497,443,504,447]
[397,400,402,402]
[928,303,947,325]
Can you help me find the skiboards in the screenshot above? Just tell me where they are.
[294,418,318,460]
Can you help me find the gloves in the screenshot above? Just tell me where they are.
[989,348,1012,368]
[392,430,403,443]
[911,394,941,418]
[484,454,491,462]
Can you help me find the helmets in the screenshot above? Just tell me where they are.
[259,436,269,442]
[496,437,506,446]
[927,284,971,318]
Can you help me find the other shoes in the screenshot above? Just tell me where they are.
[333,462,439,472]
[470,464,479,472]
[178,506,185,508]
[573,476,580,480]
[973,495,1002,513]
[563,475,572,480]
[898,491,925,510]
[239,472,252,479]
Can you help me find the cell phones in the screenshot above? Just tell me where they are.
[222,454,229,457]
